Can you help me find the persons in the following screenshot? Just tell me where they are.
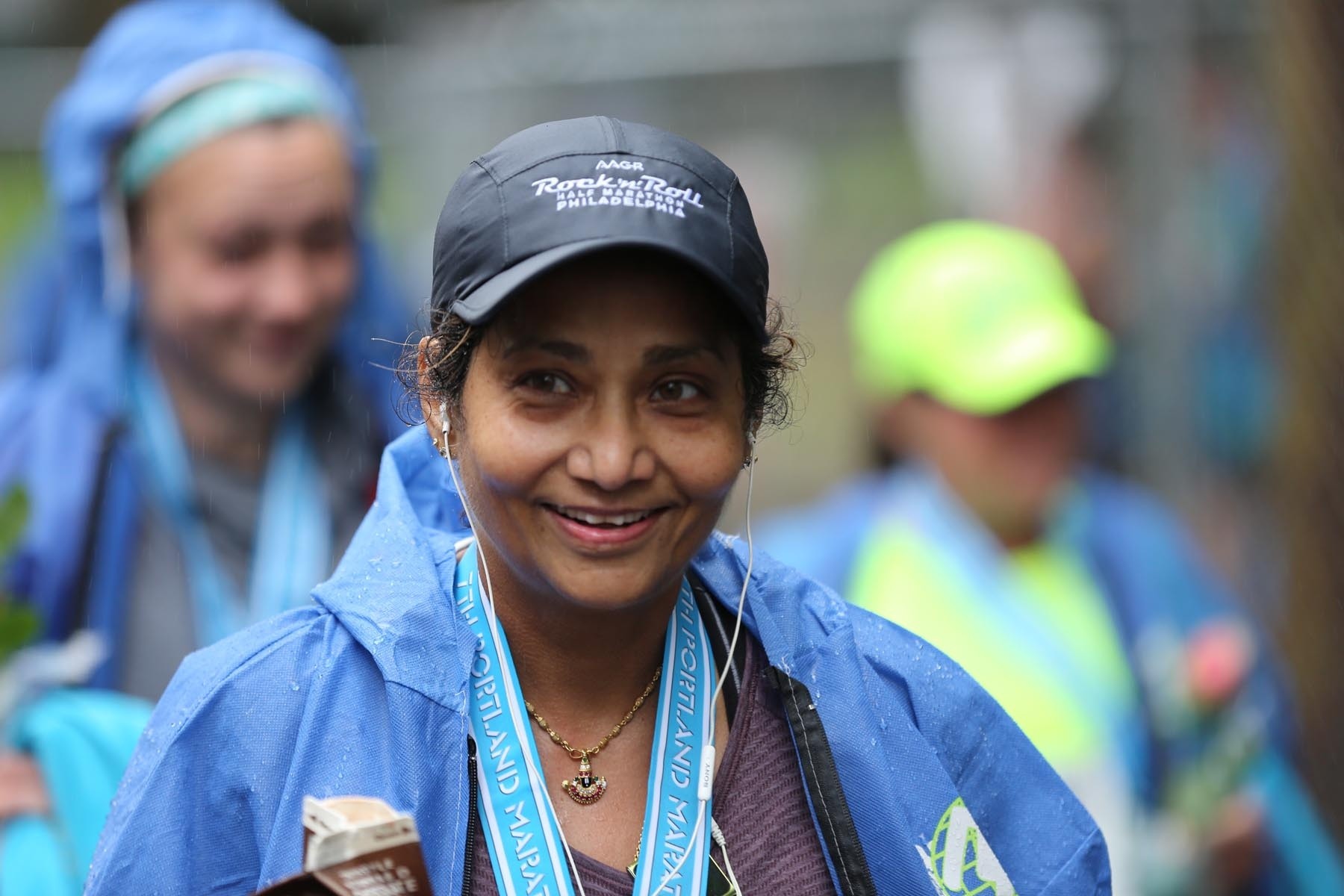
[0,0,1344,895]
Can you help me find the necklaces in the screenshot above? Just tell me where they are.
[519,662,662,805]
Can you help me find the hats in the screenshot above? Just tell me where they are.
[431,116,776,348]
[846,214,1112,417]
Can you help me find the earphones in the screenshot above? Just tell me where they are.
[745,419,756,445]
[438,401,451,434]
